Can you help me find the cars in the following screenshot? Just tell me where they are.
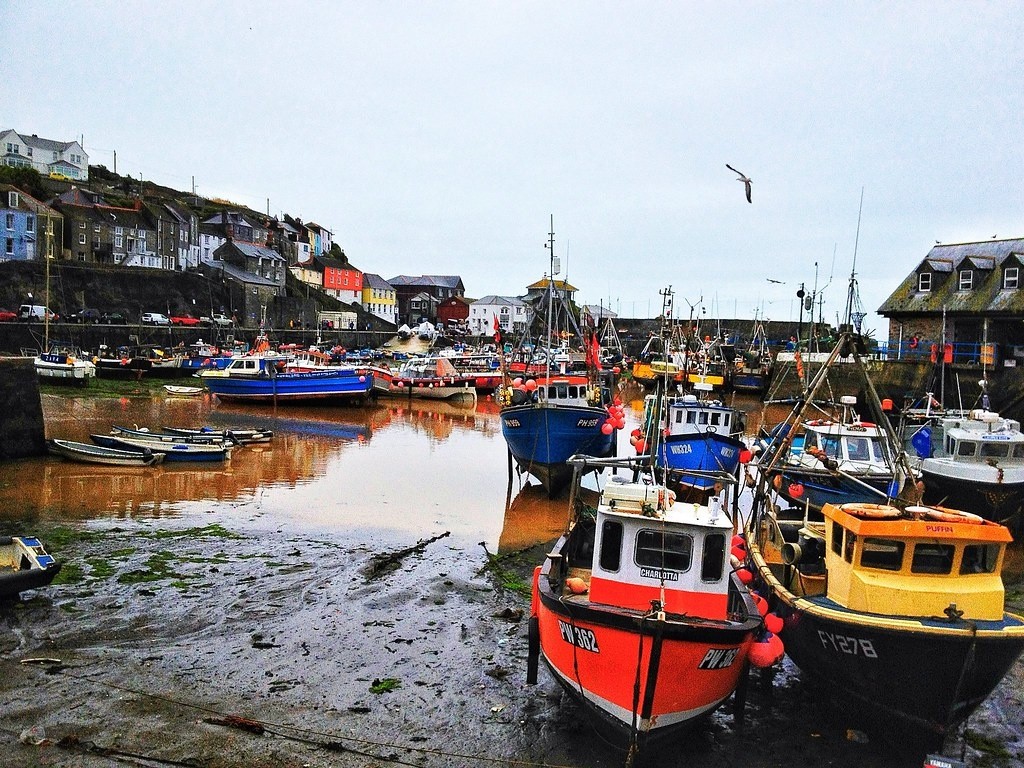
[170,312,201,327]
[101,311,129,324]
[409,325,473,337]
[0,307,18,322]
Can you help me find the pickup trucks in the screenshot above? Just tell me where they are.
[199,312,235,328]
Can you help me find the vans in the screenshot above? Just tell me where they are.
[142,311,173,326]
[18,305,56,322]
[453,342,514,353]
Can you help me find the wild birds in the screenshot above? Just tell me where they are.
[935,239,942,245]
[726,163,754,204]
[991,234,998,239]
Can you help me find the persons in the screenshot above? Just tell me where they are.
[29,309,108,325]
[231,314,237,327]
[350,322,354,331]
[297,320,302,329]
[268,318,273,328]
[290,319,294,330]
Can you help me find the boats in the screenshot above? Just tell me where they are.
[0,535,62,588]
[497,351,499,352]
[88,423,275,462]
[163,383,204,396]
[53,436,166,467]
[500,268,1024,736]
[97,230,774,398]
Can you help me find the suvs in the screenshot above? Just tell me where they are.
[63,308,102,324]
[317,318,334,330]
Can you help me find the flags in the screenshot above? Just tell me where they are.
[583,330,603,369]
[493,312,501,344]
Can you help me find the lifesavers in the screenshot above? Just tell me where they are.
[841,502,902,519]
[926,506,983,524]
[909,337,917,348]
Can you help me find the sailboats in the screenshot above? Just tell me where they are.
[32,208,97,379]
